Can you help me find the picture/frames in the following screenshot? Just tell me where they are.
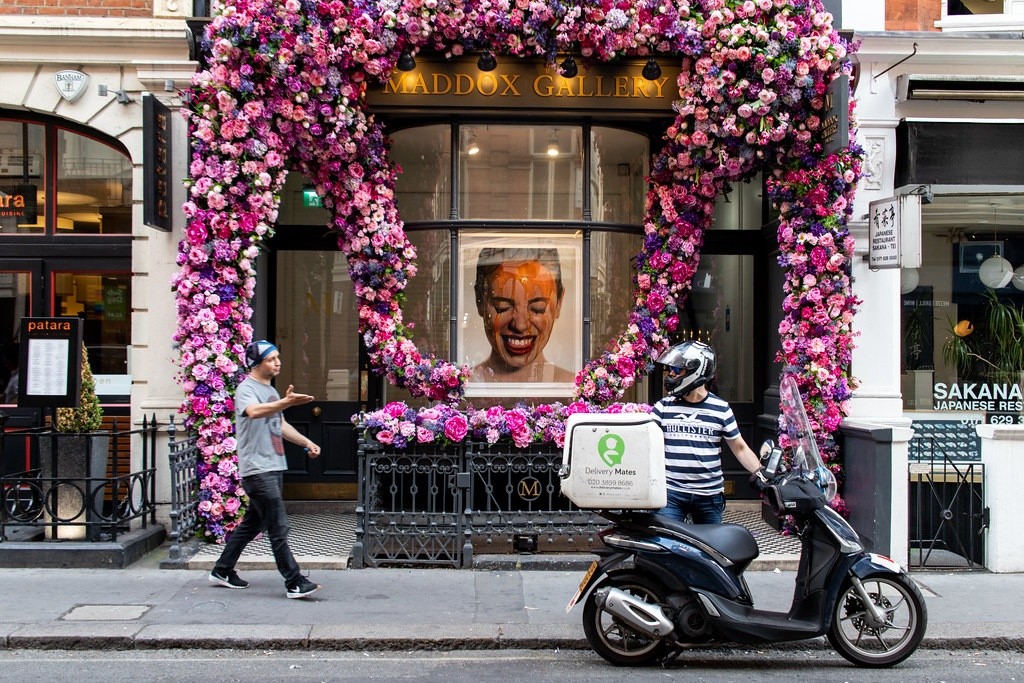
[447,232,582,389]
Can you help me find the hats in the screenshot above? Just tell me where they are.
[245,340,278,369]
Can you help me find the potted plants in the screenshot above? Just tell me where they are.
[40,341,109,541]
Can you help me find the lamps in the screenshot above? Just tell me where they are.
[560,47,578,78]
[165,79,186,96]
[397,45,416,71]
[97,84,127,103]
[901,268,919,294]
[642,44,661,80]
[477,47,497,70]
[979,200,1024,290]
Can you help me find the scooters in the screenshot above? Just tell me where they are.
[565,376,927,668]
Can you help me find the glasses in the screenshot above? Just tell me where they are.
[667,365,686,375]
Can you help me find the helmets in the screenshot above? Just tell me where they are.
[664,340,716,397]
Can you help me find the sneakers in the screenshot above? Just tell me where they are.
[209,567,249,588]
[287,576,321,599]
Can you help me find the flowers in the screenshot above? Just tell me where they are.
[166,0,868,540]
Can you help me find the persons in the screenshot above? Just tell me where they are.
[647,341,769,528]
[465,249,577,383]
[209,340,322,599]
